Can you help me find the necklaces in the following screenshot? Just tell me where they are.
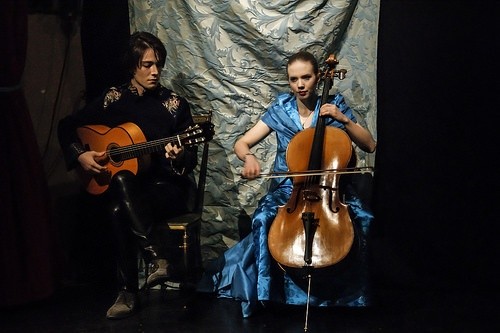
[302,109,313,129]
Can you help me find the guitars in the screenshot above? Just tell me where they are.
[78,121,215,195]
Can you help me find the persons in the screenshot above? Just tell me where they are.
[212,51,377,318]
[57,32,198,319]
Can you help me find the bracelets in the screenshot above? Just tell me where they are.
[243,152,257,157]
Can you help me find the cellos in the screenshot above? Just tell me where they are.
[268,54,355,268]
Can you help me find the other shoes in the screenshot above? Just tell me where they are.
[106,288,138,320]
[147,256,170,285]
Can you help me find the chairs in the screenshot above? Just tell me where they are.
[154,109,212,292]
